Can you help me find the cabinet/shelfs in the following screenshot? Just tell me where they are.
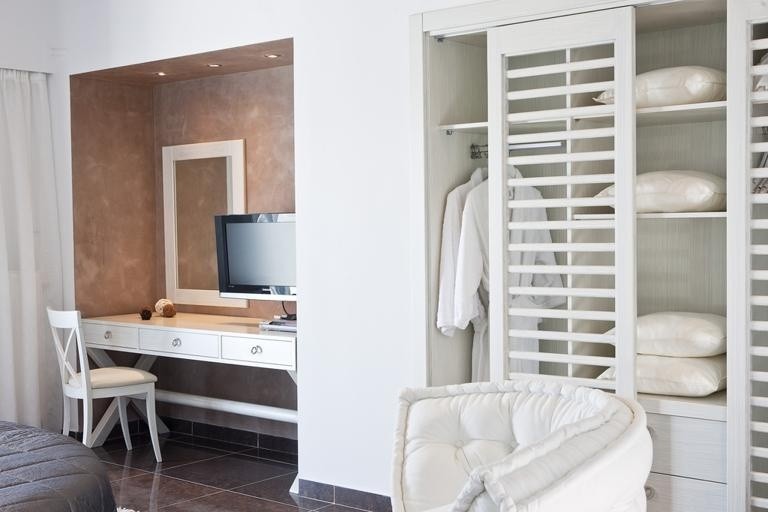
[410,0,768,512]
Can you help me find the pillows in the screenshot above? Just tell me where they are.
[591,65,727,108]
[592,173,726,212]
[595,312,726,396]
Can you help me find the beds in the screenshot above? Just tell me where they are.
[0,422,118,512]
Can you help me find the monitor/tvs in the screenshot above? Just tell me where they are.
[214,212,297,301]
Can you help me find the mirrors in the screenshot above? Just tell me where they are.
[162,139,248,308]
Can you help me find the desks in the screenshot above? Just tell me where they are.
[84,312,300,494]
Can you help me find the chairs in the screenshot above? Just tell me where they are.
[47,306,163,463]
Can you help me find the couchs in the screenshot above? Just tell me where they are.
[392,379,653,512]
